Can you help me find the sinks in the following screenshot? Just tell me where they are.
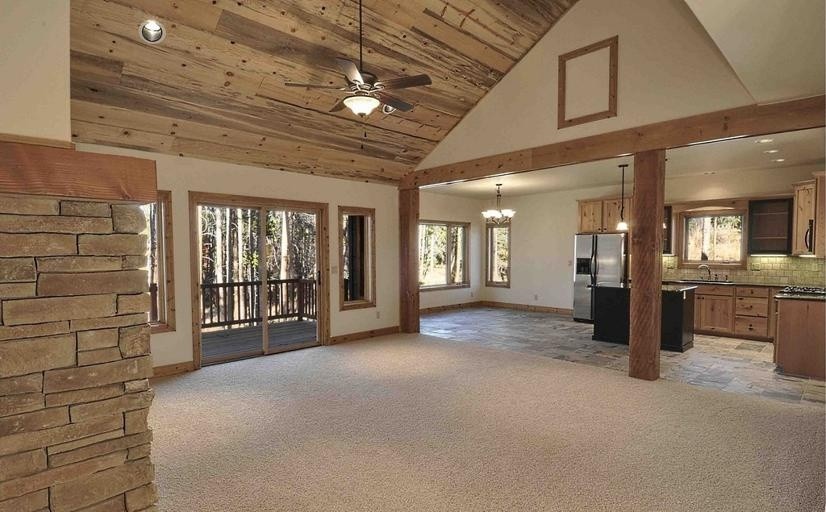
[681,279,733,283]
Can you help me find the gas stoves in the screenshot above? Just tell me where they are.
[779,286,826,296]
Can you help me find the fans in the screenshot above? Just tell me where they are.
[283,0,434,120]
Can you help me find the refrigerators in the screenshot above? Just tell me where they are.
[573,233,627,323]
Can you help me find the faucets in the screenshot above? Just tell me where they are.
[696,264,711,280]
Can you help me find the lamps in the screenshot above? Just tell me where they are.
[482,184,516,227]
[616,164,630,231]
[342,0,380,117]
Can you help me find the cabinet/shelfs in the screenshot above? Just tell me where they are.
[794,175,825,259]
[775,285,826,380]
[577,197,636,234]
[588,285,698,353]
[696,281,779,341]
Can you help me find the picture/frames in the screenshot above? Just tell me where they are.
[556,35,619,130]
